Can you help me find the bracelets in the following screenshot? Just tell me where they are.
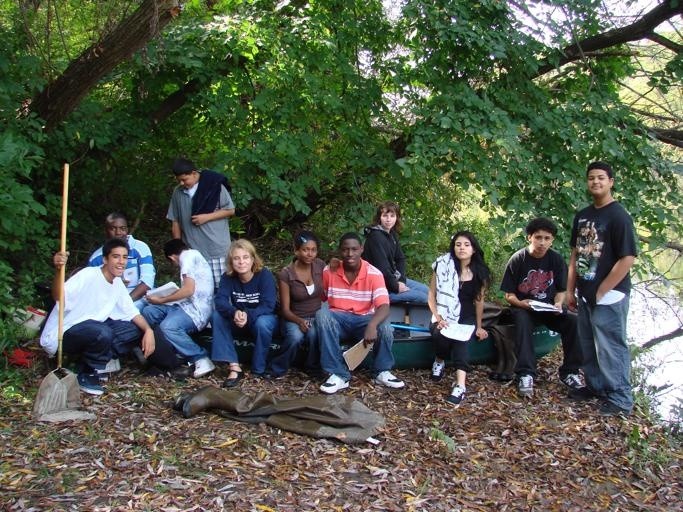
[568,289,575,292]
[438,319,443,324]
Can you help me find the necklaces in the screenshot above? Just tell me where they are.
[305,279,312,284]
[348,267,357,283]
[458,266,469,290]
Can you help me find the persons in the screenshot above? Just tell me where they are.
[166,157,233,297]
[499,218,589,399]
[428,230,491,405]
[314,232,405,394]
[39,238,157,397]
[565,162,638,416]
[88,214,155,366]
[140,237,216,379]
[210,238,280,388]
[268,230,327,381]
[362,198,431,305]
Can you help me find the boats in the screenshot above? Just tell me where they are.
[191,302,563,368]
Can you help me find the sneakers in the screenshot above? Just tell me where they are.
[598,401,630,416]
[194,356,216,378]
[517,374,534,397]
[320,373,349,394]
[445,384,466,405]
[557,368,585,389]
[567,386,600,399]
[431,359,445,381]
[375,371,405,389]
[76,358,121,395]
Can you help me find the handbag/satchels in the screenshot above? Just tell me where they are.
[146,325,177,376]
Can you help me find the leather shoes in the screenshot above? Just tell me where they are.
[224,370,244,387]
[251,372,273,379]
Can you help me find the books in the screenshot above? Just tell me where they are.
[528,299,559,312]
[144,281,186,306]
[440,324,475,342]
[342,336,374,372]
